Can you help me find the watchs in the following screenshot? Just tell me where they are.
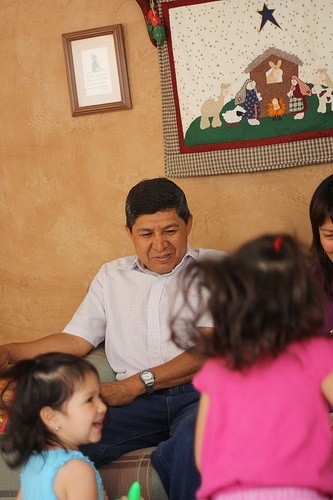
[139,369,155,394]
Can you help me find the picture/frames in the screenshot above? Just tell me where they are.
[61,23,133,118]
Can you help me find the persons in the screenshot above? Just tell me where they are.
[0,352,145,500]
[0,178,231,500]
[309,176,333,297]
[169,232,333,500]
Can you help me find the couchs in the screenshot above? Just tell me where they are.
[0,346,169,500]
